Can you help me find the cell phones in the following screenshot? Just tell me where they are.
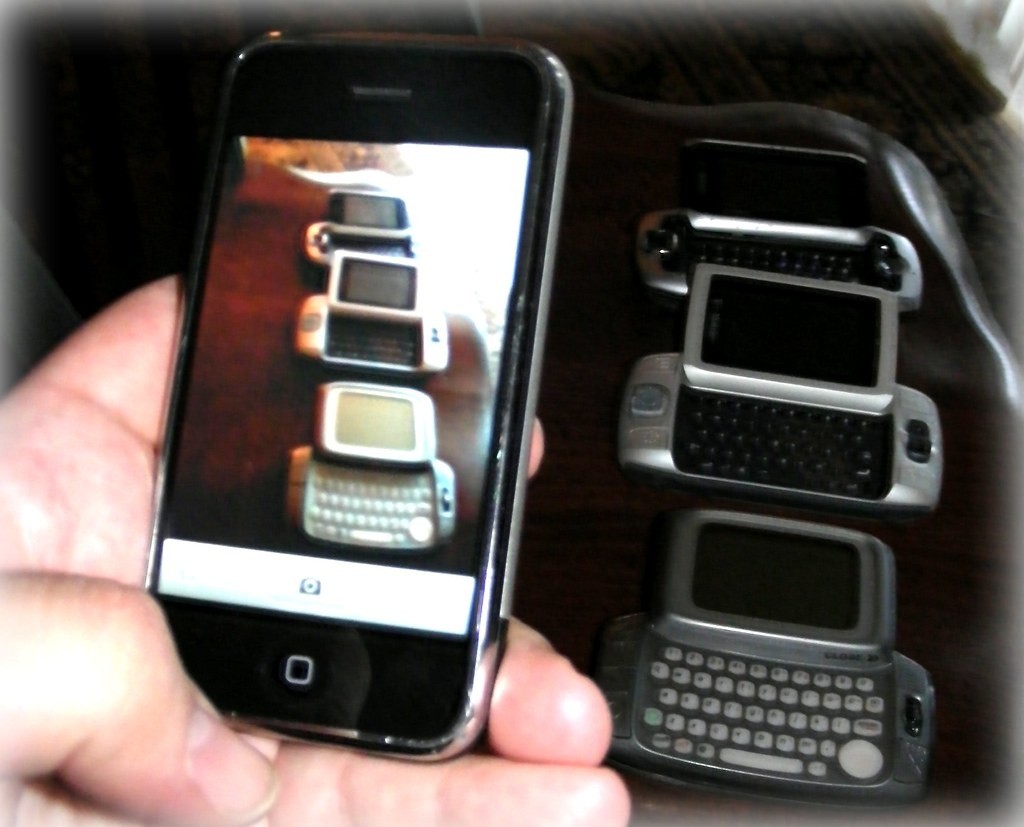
[287,187,457,553]
[143,37,575,759]
[592,139,946,810]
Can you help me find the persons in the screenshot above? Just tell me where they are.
[0,273,632,827]
